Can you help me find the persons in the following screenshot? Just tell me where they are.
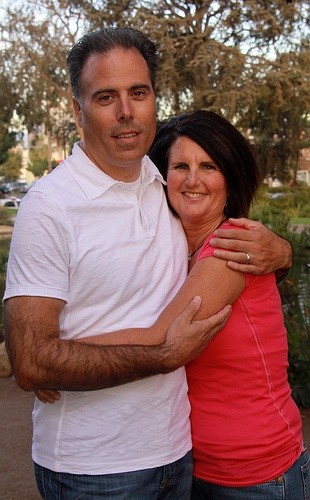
[0,25,294,500]
[30,110,310,500]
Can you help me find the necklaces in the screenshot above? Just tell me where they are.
[188,218,227,261]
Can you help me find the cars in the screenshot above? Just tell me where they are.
[0,182,12,193]
[0,196,23,218]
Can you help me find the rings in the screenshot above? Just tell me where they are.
[245,252,252,264]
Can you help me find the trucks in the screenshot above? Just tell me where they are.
[15,177,28,193]
